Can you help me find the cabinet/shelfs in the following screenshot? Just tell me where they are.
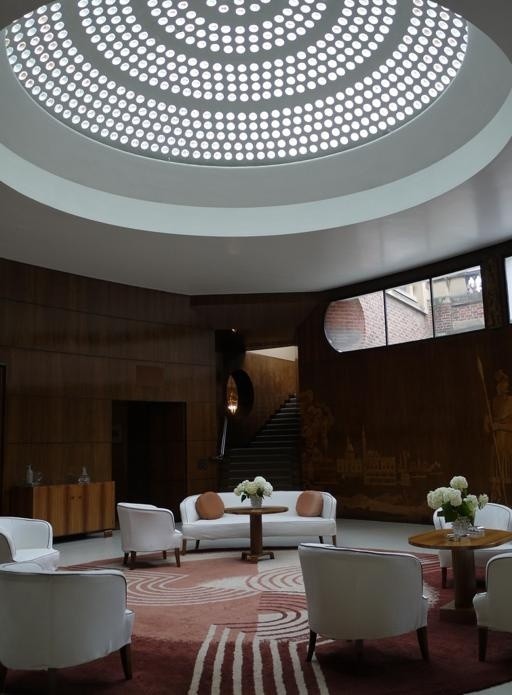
[13,480,118,538]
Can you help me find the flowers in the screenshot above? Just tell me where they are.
[426,475,489,528]
[233,475,274,502]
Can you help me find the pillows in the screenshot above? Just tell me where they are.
[195,492,225,519]
[296,490,323,517]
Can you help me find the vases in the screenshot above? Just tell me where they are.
[453,517,471,537]
[249,495,263,507]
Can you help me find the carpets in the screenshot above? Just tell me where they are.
[1,546,512,694]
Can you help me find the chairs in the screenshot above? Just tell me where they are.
[297,542,430,661]
[117,502,182,570]
[0,512,62,572]
[472,553,512,662]
[433,502,512,588]
[1,560,136,693]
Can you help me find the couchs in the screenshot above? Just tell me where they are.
[179,490,338,556]
